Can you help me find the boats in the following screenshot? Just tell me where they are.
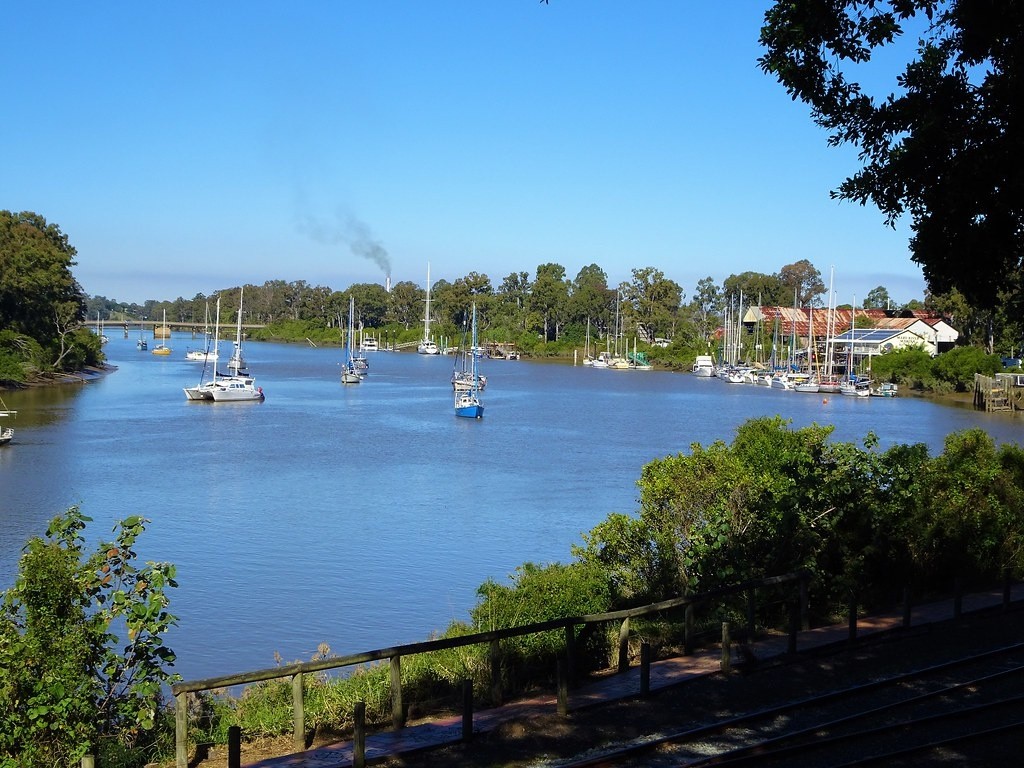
[359,330,379,353]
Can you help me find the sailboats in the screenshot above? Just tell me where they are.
[186,301,218,363]
[133,315,149,354]
[418,260,436,355]
[582,290,655,370]
[182,288,261,402]
[151,309,172,359]
[452,298,487,419]
[227,283,250,372]
[340,296,369,384]
[691,267,899,397]
